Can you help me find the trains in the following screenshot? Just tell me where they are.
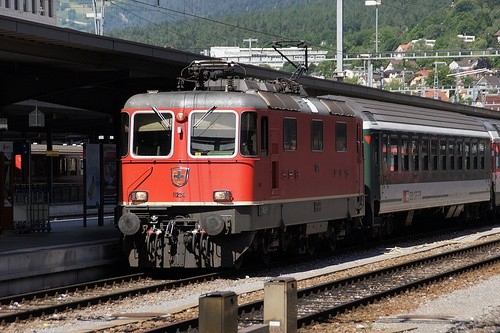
[115,61,500,279]
